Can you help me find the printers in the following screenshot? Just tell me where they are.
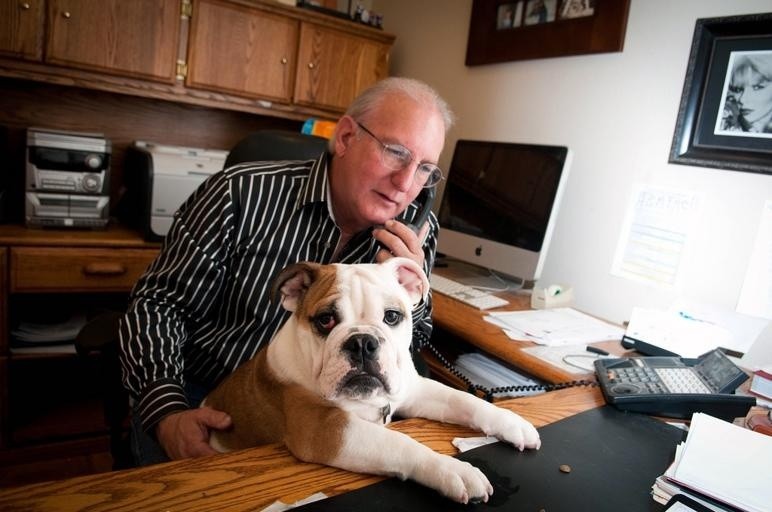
[114,141,232,238]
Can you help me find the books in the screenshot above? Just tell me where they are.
[9,319,92,356]
[652,411,771,511]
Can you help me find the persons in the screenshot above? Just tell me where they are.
[119,75,452,467]
[713,55,771,138]
[498,0,547,29]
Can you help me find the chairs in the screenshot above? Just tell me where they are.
[220,129,338,173]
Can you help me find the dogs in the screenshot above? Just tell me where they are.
[193,255,541,505]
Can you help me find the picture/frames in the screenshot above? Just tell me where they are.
[666,12,772,177]
[461,1,630,67]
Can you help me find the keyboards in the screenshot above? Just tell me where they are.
[429,273,509,310]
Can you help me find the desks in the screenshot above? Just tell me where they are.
[1,369,772,511]
[415,258,705,401]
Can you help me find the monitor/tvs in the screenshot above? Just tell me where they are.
[435,140,571,290]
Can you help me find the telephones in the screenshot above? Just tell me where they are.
[410,176,434,234]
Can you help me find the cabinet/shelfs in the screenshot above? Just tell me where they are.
[1,218,166,465]
[180,2,397,122]
[0,1,181,98]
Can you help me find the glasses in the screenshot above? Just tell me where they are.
[356,121,448,190]
